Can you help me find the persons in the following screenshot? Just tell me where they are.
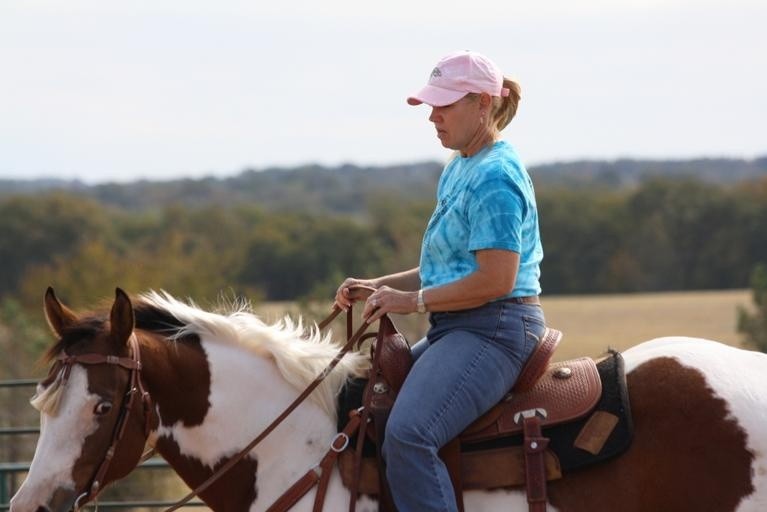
[321,46,549,511]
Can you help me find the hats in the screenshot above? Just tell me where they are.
[404,48,511,110]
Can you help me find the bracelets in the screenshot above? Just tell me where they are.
[415,288,426,317]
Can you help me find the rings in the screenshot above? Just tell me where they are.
[370,299,378,307]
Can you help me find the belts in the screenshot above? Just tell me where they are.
[499,290,543,306]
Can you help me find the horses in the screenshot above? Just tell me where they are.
[8,286,767,512]
